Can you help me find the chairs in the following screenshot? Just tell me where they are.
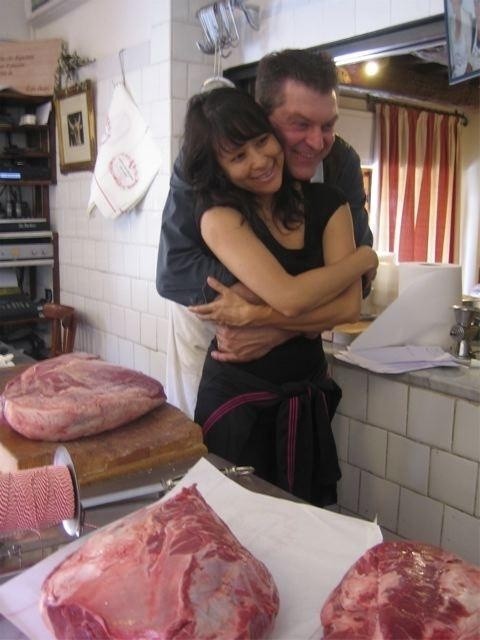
[41,302,76,358]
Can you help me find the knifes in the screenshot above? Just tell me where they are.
[82,465,258,508]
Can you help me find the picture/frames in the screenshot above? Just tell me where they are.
[24,0,63,21]
[55,79,98,175]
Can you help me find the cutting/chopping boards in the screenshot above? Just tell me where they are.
[1,347,207,488]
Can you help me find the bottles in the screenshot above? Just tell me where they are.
[373,252,400,314]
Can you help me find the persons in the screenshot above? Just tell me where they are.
[182,86,381,512]
[150,46,378,428]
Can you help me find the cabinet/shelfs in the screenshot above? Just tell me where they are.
[0,86,61,359]
